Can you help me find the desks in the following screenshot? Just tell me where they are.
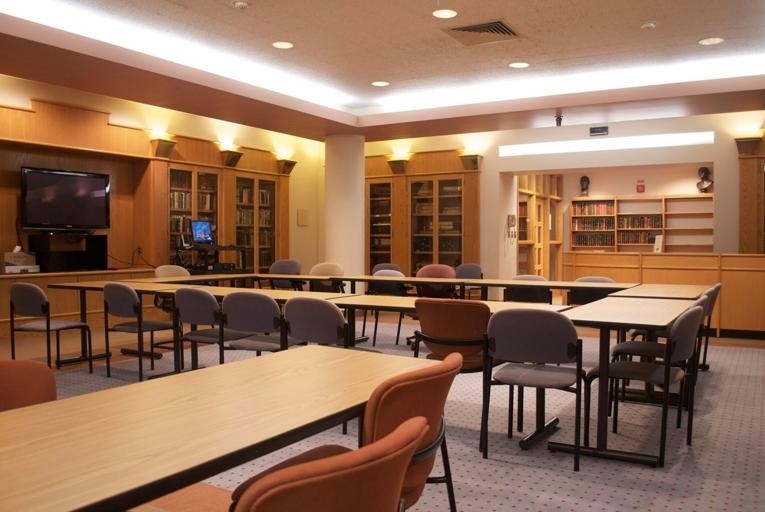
[548,297,706,467]
[0,346,445,510]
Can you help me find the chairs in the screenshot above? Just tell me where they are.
[611,282,725,372]
[228,350,462,510]
[584,306,704,466]
[224,417,432,512]
[407,299,490,452]
[2,259,643,378]
[480,308,589,471]
[607,296,704,417]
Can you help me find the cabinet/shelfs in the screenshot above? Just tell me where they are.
[569,194,616,251]
[227,144,299,290]
[616,193,665,252]
[159,132,227,268]
[664,192,716,255]
[362,153,408,295]
[409,150,484,304]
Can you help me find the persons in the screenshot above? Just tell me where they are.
[580,176,590,192]
[696,167,713,192]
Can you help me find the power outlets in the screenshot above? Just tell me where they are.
[136,244,143,257]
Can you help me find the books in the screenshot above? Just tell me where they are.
[572,202,662,246]
[170,190,214,232]
[237,185,275,273]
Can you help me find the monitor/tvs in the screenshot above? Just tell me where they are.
[191,220,213,242]
[20,166,110,229]
